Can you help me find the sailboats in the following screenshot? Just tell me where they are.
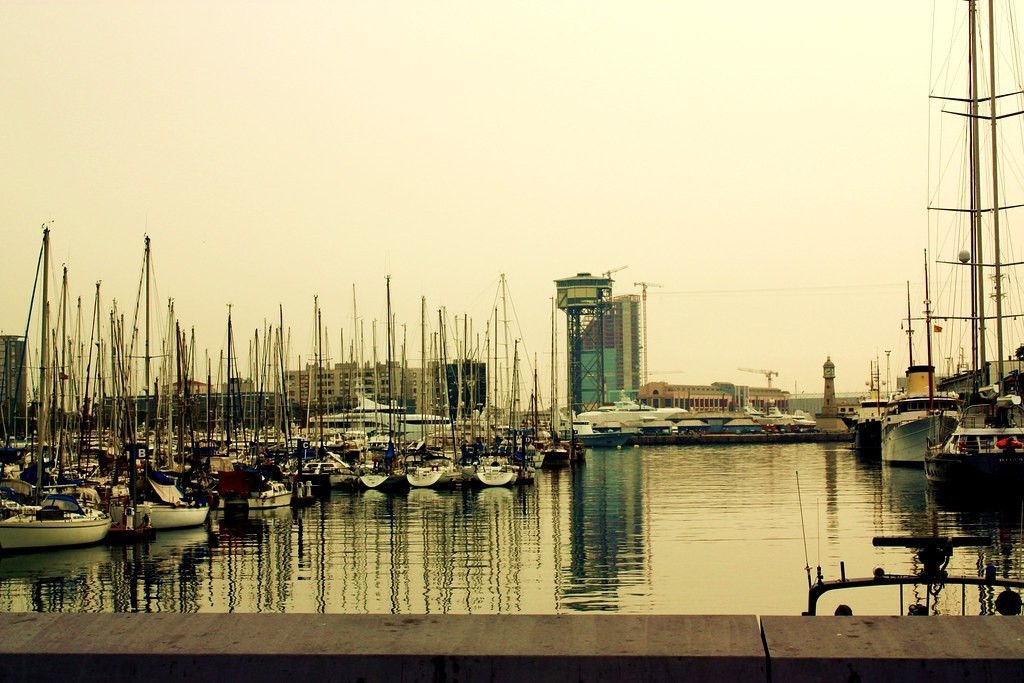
[0,219,586,549]
[924,0,1024,502]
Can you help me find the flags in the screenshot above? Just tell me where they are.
[934,325,942,332]
[59,372,68,379]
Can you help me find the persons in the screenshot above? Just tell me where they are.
[459,440,484,466]
[959,438,966,454]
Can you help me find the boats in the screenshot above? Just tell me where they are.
[881,248,966,461]
[856,350,893,449]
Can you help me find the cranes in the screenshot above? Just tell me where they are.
[634,282,663,385]
[737,367,779,388]
[601,265,628,302]
